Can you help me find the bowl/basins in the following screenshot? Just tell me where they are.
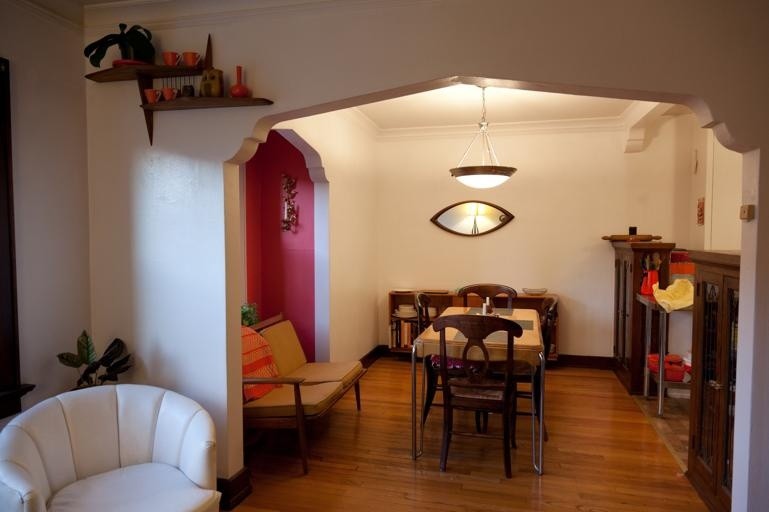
[522,288,547,295]
[648,353,692,382]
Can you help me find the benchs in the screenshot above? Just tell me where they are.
[240,313,368,476]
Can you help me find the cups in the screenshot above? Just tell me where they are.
[629,227,637,235]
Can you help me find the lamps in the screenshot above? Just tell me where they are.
[448,84,518,189]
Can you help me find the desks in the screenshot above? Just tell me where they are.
[411,307,546,474]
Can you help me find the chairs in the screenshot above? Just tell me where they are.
[483,301,558,442]
[458,283,518,308]
[0,384,222,512]
[418,306,482,433]
[433,315,523,478]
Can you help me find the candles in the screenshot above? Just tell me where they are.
[284,201,287,219]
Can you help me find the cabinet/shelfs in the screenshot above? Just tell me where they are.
[610,241,675,396]
[86,33,274,146]
[684,250,741,512]
[389,289,559,361]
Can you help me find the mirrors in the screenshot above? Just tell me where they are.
[430,200,514,236]
